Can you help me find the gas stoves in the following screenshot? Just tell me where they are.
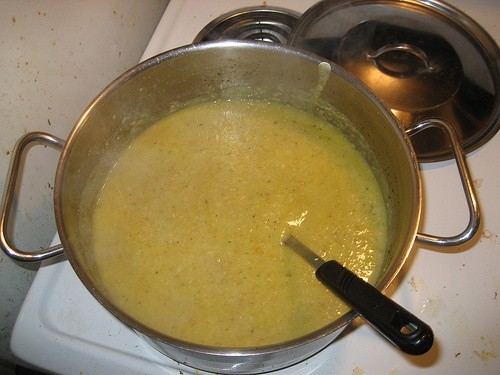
[192,6,303,46]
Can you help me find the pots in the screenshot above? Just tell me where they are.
[0,39,483,375]
